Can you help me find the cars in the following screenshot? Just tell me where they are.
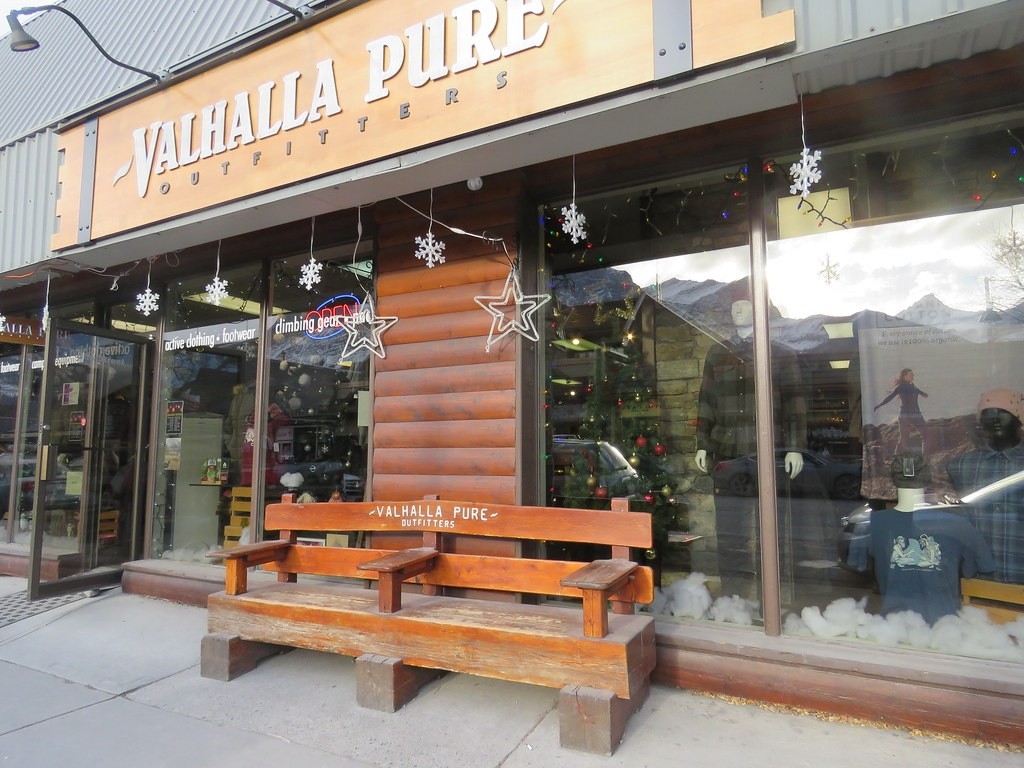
[0,450,74,524]
[836,466,1023,591]
[21,455,129,535]
[710,445,863,504]
[551,433,697,550]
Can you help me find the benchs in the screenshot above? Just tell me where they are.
[199,494,659,756]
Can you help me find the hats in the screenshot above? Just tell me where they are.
[731,289,751,303]
[891,453,933,488]
[976,387,1024,425]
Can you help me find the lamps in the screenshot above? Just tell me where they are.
[7,5,164,90]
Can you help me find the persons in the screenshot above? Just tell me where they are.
[944,389,1024,585]
[872,369,932,458]
[222,381,294,484]
[848,452,993,632]
[694,287,807,618]
[0,440,120,520]
